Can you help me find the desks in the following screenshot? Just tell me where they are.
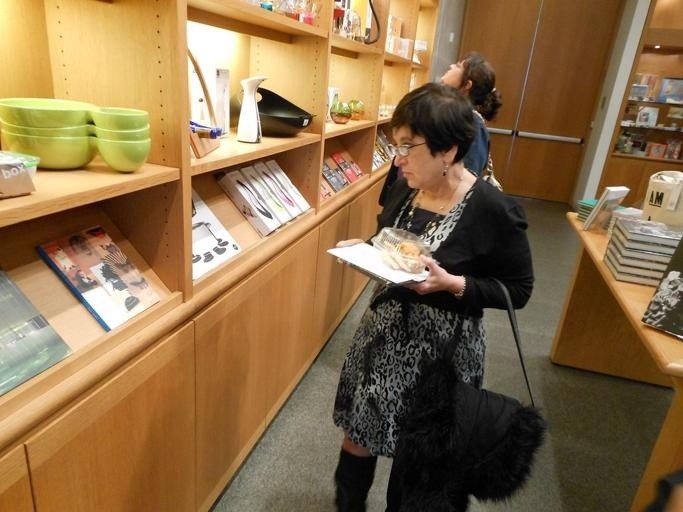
[548,212,683,512]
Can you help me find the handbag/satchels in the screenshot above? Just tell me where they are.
[641,170,683,229]
[402,356,545,502]
[472,161,503,191]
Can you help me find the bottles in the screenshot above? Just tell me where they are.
[237,73,267,144]
[326,85,366,125]
[250,0,362,42]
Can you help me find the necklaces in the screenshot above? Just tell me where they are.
[407,175,460,240]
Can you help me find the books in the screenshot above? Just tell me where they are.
[190,184,240,279]
[602,206,682,287]
[574,199,627,230]
[581,185,630,231]
[640,235,683,340]
[0,266,70,396]
[35,223,160,332]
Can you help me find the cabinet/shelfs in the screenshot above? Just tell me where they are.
[594,1,683,209]
[1,0,441,512]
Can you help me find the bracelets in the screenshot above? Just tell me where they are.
[454,275,465,298]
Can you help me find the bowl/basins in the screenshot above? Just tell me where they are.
[0,97,153,172]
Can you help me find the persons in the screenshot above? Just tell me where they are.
[333,81,533,510]
[378,53,502,224]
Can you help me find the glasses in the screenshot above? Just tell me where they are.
[386,142,427,157]
